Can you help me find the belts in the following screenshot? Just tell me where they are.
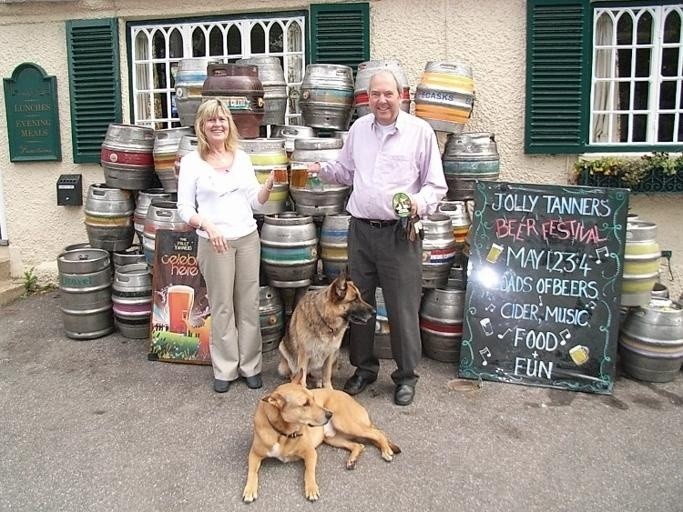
[360,218,396,234]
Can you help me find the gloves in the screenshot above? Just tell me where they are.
[392,214,423,242]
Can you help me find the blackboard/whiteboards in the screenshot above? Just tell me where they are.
[457,180,631,396]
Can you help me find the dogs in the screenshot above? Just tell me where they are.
[241,367,402,504]
[276,263,378,391]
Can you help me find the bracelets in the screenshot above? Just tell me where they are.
[264,180,273,191]
[197,221,204,231]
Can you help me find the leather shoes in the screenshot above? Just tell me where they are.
[245,374,262,388]
[214,379,228,391]
[394,384,414,405]
[342,374,373,395]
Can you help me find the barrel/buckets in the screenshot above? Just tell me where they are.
[54,55,683,384]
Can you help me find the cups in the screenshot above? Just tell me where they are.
[167,289,193,335]
[271,165,289,188]
[568,344,591,365]
[289,162,311,190]
[480,317,494,337]
[485,243,504,264]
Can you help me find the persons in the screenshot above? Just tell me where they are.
[175,97,277,394]
[304,69,449,406]
